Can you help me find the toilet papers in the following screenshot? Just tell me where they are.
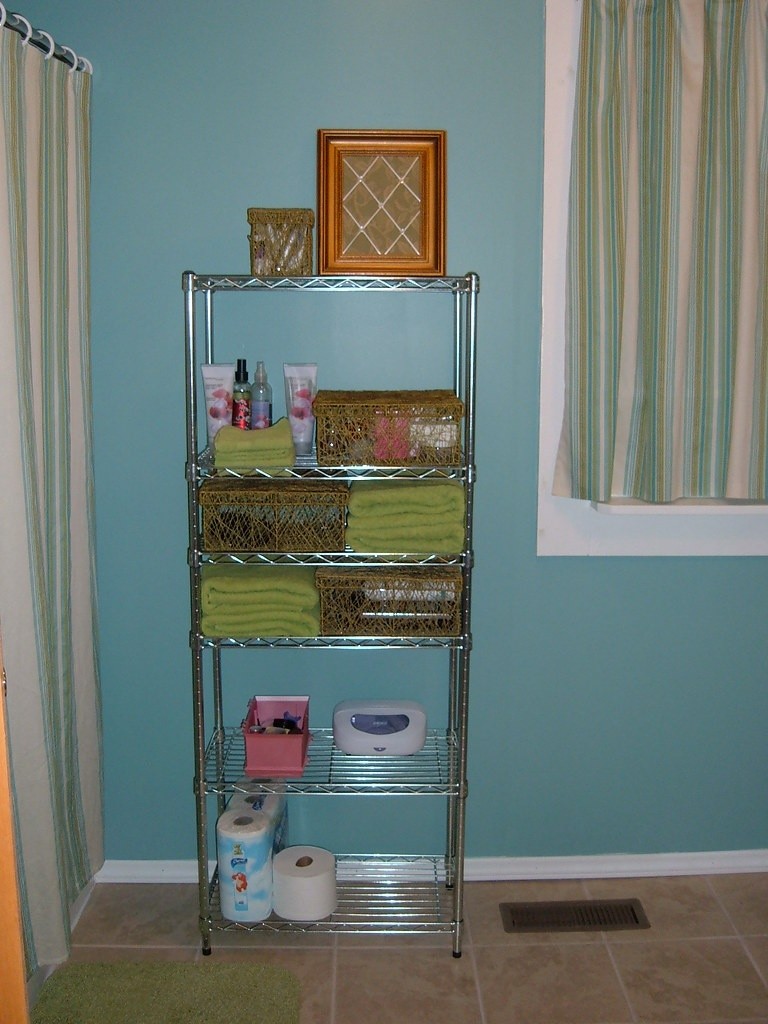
[271,841,341,922]
[215,775,287,922]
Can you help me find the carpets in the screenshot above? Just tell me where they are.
[29,963,304,1024]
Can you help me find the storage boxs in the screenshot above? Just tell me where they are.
[241,695,311,778]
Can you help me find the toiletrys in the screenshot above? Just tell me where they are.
[200,358,318,456]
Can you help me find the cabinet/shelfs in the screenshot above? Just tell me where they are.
[181,268,477,960]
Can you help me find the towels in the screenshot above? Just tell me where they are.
[198,555,323,639]
[213,416,296,478]
[343,469,466,557]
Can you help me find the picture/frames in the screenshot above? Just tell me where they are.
[317,127,446,277]
